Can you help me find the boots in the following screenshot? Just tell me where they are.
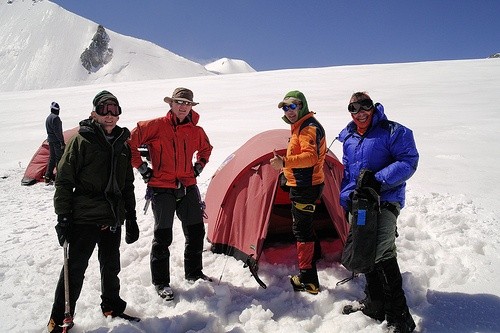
[375,257,416,333]
[341,270,386,323]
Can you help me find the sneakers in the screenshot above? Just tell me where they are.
[290,275,320,295]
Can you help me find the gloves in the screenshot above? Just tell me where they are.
[193,165,201,178]
[55,222,72,247]
[141,167,153,183]
[125,219,139,244]
[61,143,66,152]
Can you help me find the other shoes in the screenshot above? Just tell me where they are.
[44,176,55,183]
[156,283,175,301]
[102,309,141,322]
[41,318,75,333]
[185,271,213,283]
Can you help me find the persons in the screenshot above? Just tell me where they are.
[47,90,141,333]
[270,91,326,295]
[130,88,213,301]
[339,91,419,333]
[45,102,66,183]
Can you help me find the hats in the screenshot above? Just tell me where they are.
[50,102,60,115]
[92,90,120,107]
[164,87,199,106]
[278,97,303,109]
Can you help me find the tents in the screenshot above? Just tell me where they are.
[24,127,80,182]
[204,129,359,278]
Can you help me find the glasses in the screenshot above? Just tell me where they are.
[347,99,373,114]
[171,100,193,106]
[95,105,122,117]
[282,103,301,112]
